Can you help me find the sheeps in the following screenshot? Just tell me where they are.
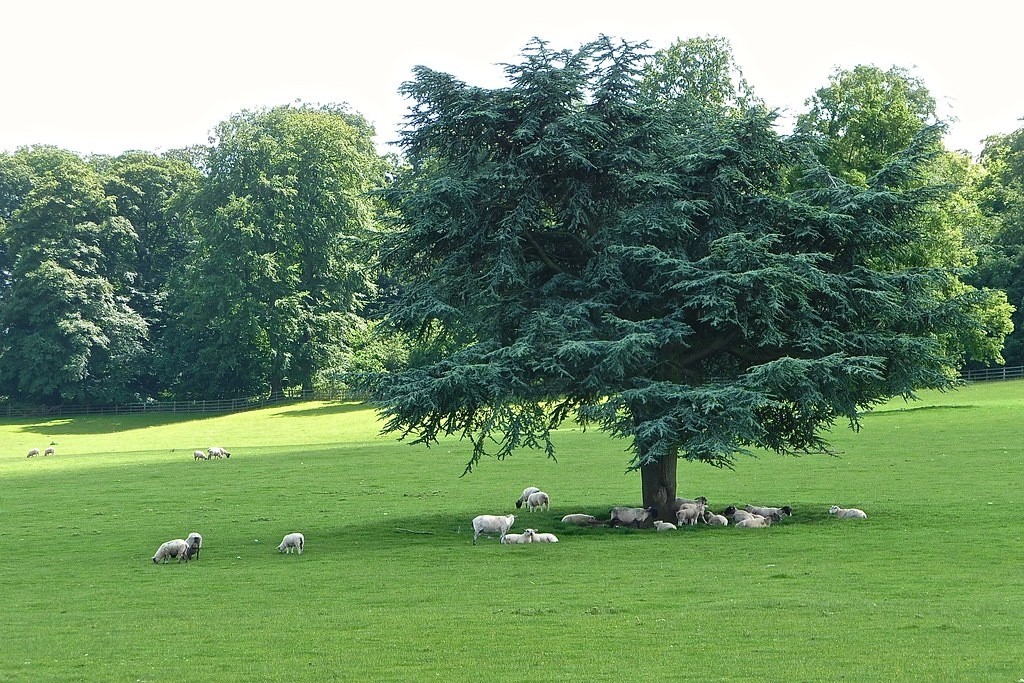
[514,487,550,513]
[44,448,55,456]
[193,447,231,461]
[501,528,559,544]
[471,513,519,546]
[653,496,793,533]
[151,533,202,565]
[27,448,40,458]
[609,506,659,530]
[561,513,596,525]
[829,505,868,519]
[275,532,305,555]
